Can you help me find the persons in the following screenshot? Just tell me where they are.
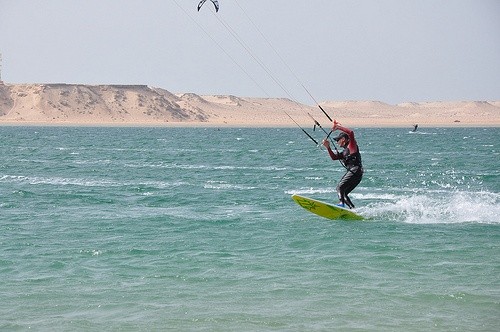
[323,125,363,209]
[412,124,418,132]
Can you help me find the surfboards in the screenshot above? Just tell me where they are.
[290,193,364,220]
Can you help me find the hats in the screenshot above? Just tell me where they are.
[333,132,349,141]
